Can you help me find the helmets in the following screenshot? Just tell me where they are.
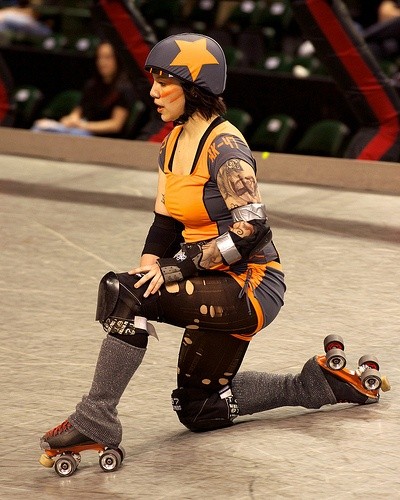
[144,32,228,94]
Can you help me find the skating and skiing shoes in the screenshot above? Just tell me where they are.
[39,419,126,477]
[315,333,391,405]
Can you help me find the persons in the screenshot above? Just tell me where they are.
[39,32,391,477]
[62,41,136,139]
[0,0,48,36]
